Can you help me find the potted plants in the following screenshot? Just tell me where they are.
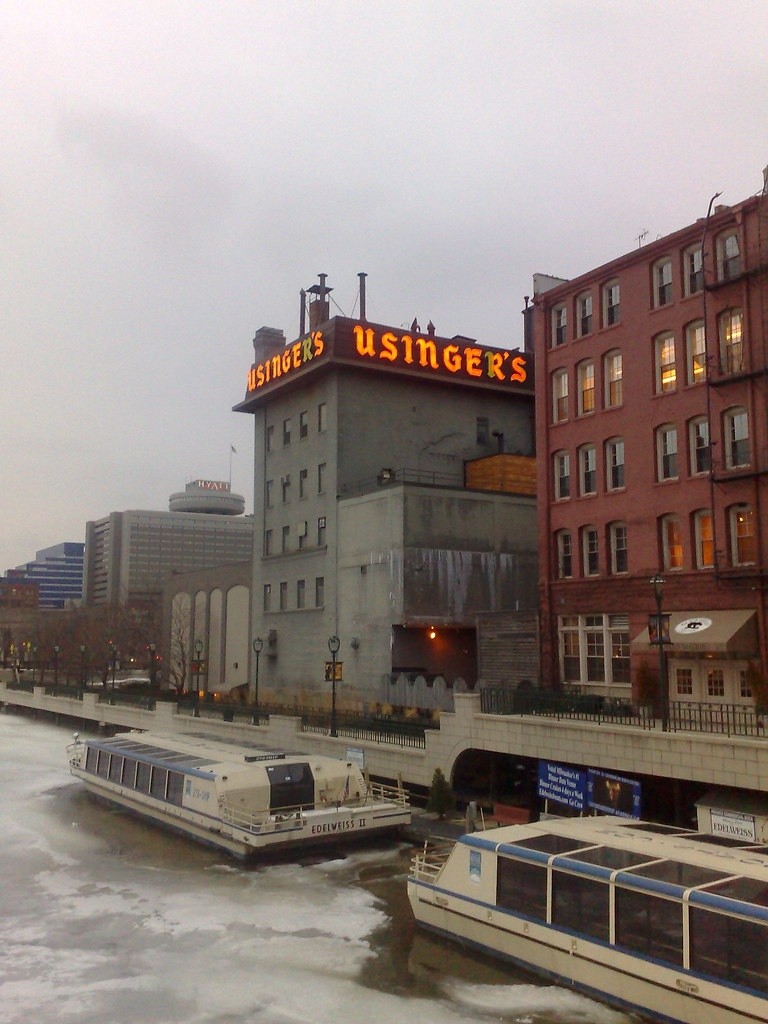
[631,661,663,720]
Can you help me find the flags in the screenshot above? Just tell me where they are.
[337,776,350,804]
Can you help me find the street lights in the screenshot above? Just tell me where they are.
[32,646,38,693]
[79,644,86,700]
[193,640,203,718]
[110,644,116,705]
[327,636,340,737]
[150,641,156,711]
[648,569,672,732]
[53,644,61,696]
[253,637,263,726]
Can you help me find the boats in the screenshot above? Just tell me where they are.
[64,733,413,863]
[406,808,768,1024]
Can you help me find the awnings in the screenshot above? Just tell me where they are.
[629,609,758,654]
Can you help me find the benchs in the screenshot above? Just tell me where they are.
[485,804,531,828]
